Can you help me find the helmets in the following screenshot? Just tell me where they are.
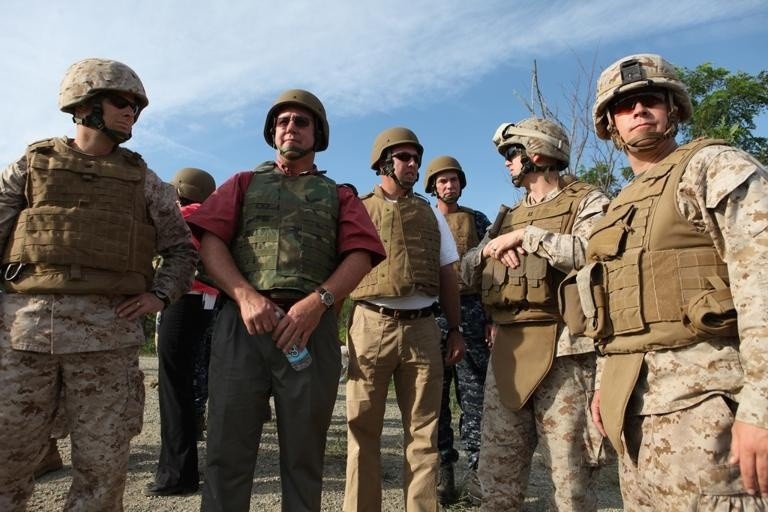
[425,156,466,192]
[170,167,215,202]
[59,59,149,112]
[492,119,569,171]
[592,53,694,139]
[264,90,329,152]
[370,129,422,170]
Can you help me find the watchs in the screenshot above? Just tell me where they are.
[316,287,337,313]
[447,325,464,332]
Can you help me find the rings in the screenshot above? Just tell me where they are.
[136,301,142,307]
[491,249,497,252]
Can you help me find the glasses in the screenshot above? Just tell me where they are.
[504,145,523,161]
[393,152,420,163]
[104,91,140,114]
[272,118,317,129]
[610,94,668,115]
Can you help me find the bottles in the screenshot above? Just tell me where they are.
[271,310,314,372]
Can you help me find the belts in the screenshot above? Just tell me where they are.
[354,299,437,319]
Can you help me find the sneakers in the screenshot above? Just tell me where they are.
[141,481,196,495]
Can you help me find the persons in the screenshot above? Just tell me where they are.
[145,168,216,496]
[1,57,198,511]
[578,53,768,512]
[344,128,468,512]
[462,118,617,512]
[29,436,63,481]
[153,255,213,441]
[187,88,385,510]
[423,156,491,504]
[264,405,271,419]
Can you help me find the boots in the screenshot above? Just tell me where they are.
[459,468,482,504]
[436,463,454,503]
